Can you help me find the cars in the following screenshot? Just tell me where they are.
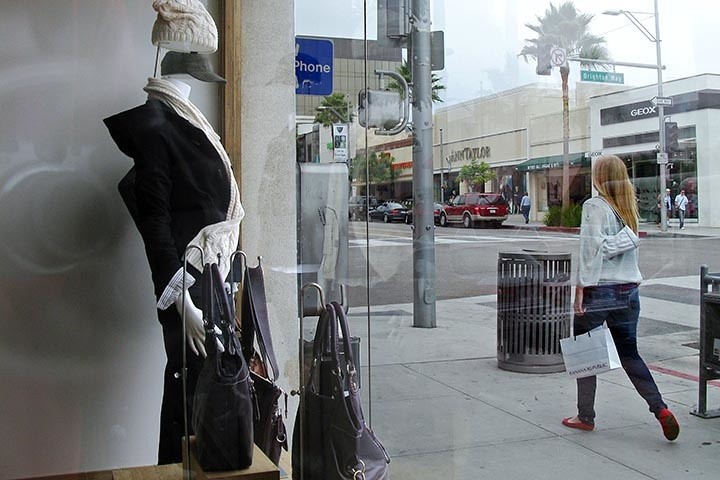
[369,201,412,223]
[405,203,444,227]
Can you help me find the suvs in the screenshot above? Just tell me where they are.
[348,196,378,220]
[440,191,509,228]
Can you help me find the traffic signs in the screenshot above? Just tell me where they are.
[649,96,672,107]
[657,152,668,164]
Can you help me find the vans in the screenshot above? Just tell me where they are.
[600,159,697,195]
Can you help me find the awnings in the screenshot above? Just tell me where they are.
[514,153,591,175]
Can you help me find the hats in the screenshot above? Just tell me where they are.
[161,50,228,85]
[666,188,671,192]
[151,1,219,54]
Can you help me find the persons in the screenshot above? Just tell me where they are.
[656,194,669,229]
[514,185,520,214]
[666,188,672,227]
[101,0,246,465]
[675,189,689,230]
[520,192,530,224]
[449,194,455,203]
[562,155,680,441]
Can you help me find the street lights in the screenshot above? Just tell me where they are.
[318,94,350,183]
[602,0,667,231]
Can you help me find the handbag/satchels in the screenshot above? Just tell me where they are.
[193,265,255,473]
[675,206,679,211]
[237,264,289,466]
[292,304,391,480]
[593,196,642,259]
[558,310,623,380]
[653,208,657,214]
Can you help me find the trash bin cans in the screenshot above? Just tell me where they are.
[497,248,572,374]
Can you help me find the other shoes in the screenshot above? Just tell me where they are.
[562,417,594,430]
[527,219,529,224]
[681,226,685,230]
[660,409,680,441]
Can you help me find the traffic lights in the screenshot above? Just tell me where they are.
[665,122,679,152]
[536,38,553,75]
